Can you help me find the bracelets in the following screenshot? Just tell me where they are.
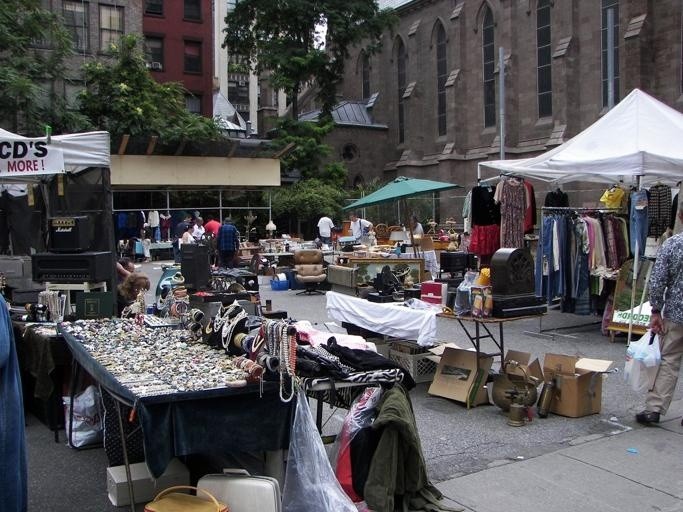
[650,309,662,314]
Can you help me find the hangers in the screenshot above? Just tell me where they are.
[542,206,624,220]
[607,175,683,192]
[499,174,523,184]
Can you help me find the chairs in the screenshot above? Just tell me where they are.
[294,250,328,296]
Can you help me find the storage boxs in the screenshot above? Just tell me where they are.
[428,346,615,417]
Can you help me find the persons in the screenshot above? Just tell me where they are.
[348,211,374,244]
[115,255,135,287]
[634,201,683,429]
[118,211,240,268]
[1,292,29,511]
[317,210,344,244]
[409,215,423,237]
[373,264,400,295]
[117,271,151,318]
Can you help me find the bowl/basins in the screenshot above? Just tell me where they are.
[380,254,391,258]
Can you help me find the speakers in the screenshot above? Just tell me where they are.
[46,216,96,253]
[180,245,208,290]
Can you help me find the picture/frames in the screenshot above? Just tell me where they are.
[350,258,424,293]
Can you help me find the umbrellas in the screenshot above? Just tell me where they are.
[342,175,465,259]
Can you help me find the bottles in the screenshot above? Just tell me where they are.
[285,243,289,252]
[21,314,43,322]
[537,380,556,419]
[25,302,47,314]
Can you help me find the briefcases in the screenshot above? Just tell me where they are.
[198,469,282,512]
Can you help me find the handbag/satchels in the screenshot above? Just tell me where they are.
[143,485,228,512]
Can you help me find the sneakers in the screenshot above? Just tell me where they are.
[636,410,660,423]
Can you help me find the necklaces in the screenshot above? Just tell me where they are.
[205,300,297,405]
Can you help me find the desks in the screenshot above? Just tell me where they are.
[434,309,550,378]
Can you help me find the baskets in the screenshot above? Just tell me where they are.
[388,348,438,382]
[270,279,289,291]
